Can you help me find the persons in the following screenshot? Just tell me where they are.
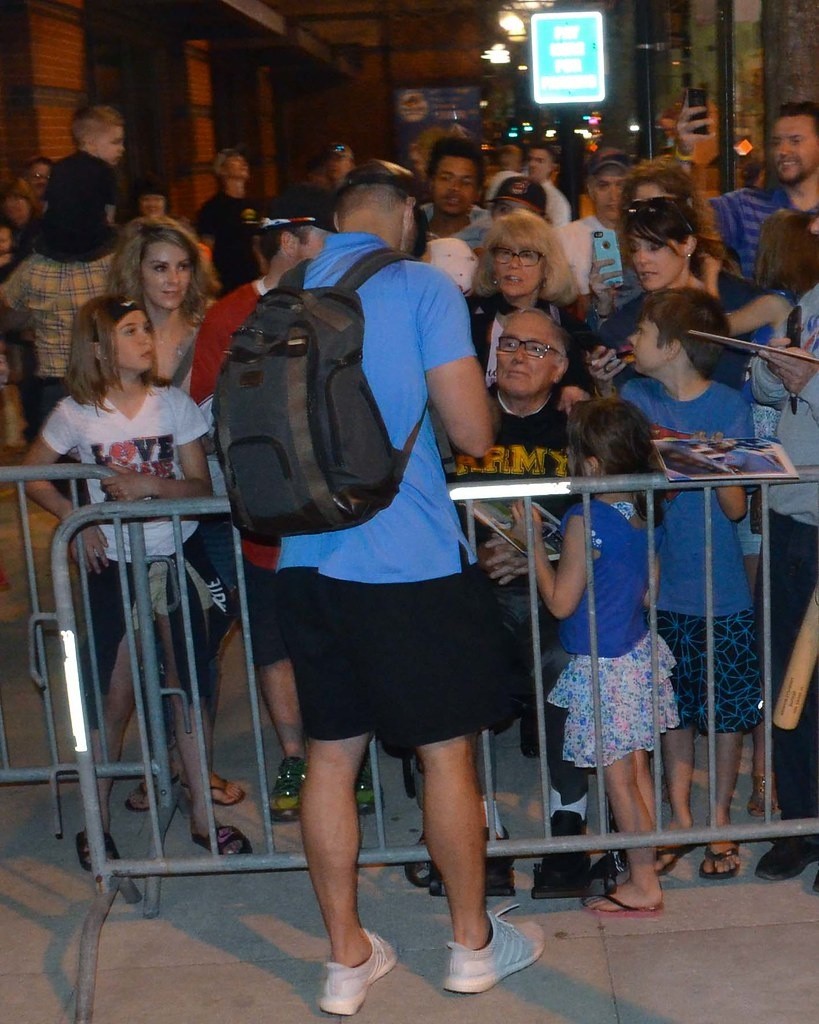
[410,126,819,915]
[269,160,546,1014]
[0,108,383,872]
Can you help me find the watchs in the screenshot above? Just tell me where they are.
[594,302,610,318]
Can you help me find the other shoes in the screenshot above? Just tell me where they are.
[747,773,777,818]
[406,836,435,889]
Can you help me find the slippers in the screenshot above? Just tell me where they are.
[126,772,184,811]
[582,895,666,917]
[181,772,244,806]
[193,826,253,856]
[656,844,697,875]
[75,831,120,873]
[700,845,742,880]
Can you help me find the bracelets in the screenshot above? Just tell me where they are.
[675,146,696,160]
[257,276,267,296]
[604,366,611,373]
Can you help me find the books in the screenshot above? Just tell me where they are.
[460,498,560,562]
[688,329,819,365]
[653,436,800,481]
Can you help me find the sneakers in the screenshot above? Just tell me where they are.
[543,810,586,871]
[757,837,819,882]
[319,926,398,1013]
[356,760,385,816]
[269,756,305,821]
[444,907,545,992]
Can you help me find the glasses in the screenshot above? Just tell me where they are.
[498,336,563,359]
[622,196,693,233]
[490,245,545,267]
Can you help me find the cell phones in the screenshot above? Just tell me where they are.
[787,306,802,350]
[591,229,624,284]
[687,88,708,135]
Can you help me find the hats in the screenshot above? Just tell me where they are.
[486,175,547,212]
[257,185,338,234]
[590,152,633,174]
[335,159,429,257]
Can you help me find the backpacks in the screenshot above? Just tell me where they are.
[214,248,428,545]
